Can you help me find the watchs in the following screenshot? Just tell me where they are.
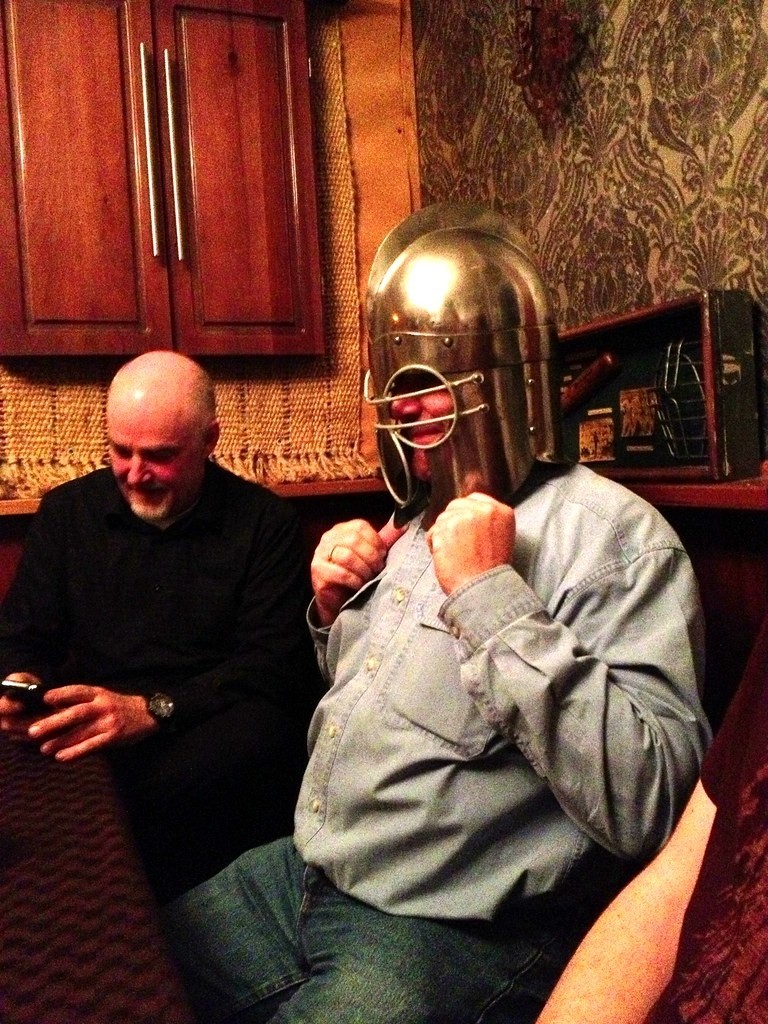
[142,689,175,728]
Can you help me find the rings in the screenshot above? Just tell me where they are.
[329,544,339,561]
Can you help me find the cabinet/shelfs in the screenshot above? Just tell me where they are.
[0,0,325,358]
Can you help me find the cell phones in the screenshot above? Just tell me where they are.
[0,680,49,716]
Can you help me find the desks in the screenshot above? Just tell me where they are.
[1,730,200,1024]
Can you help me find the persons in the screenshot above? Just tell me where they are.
[142,198,709,1024]
[0,349,337,979]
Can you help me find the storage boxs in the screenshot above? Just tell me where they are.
[554,285,761,482]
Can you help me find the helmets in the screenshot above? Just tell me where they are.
[363,201,556,532]
